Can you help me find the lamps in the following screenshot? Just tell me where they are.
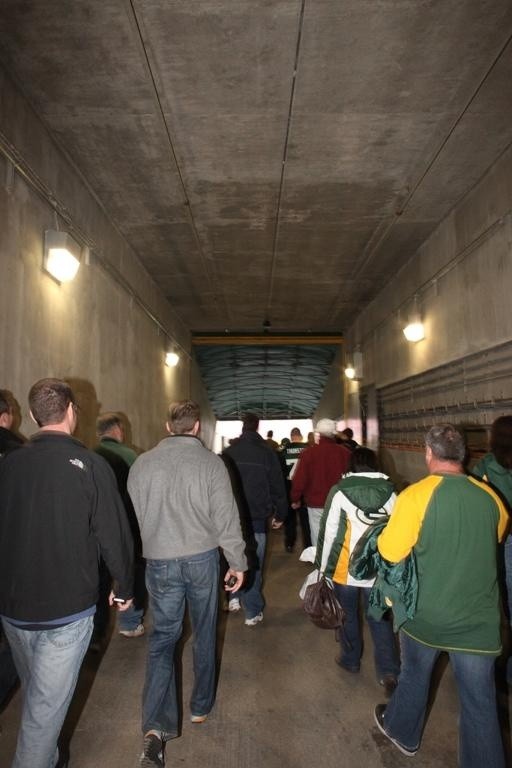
[165,346,180,367]
[44,206,84,286]
[400,293,426,344]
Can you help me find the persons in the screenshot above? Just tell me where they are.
[91,413,147,637]
[313,446,402,694]
[126,398,251,767]
[370,422,509,768]
[1,389,25,459]
[1,377,137,767]
[217,413,359,625]
[467,416,511,638]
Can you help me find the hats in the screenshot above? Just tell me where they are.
[316,418,336,433]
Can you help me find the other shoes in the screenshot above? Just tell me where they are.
[374,704,417,756]
[382,677,397,698]
[335,653,360,675]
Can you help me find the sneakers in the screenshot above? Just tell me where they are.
[222,600,241,613]
[120,624,143,638]
[190,713,207,723]
[140,731,163,768]
[243,610,266,627]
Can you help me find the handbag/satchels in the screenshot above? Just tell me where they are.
[303,584,345,631]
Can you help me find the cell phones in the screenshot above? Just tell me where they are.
[114,598,125,607]
[227,576,237,587]
